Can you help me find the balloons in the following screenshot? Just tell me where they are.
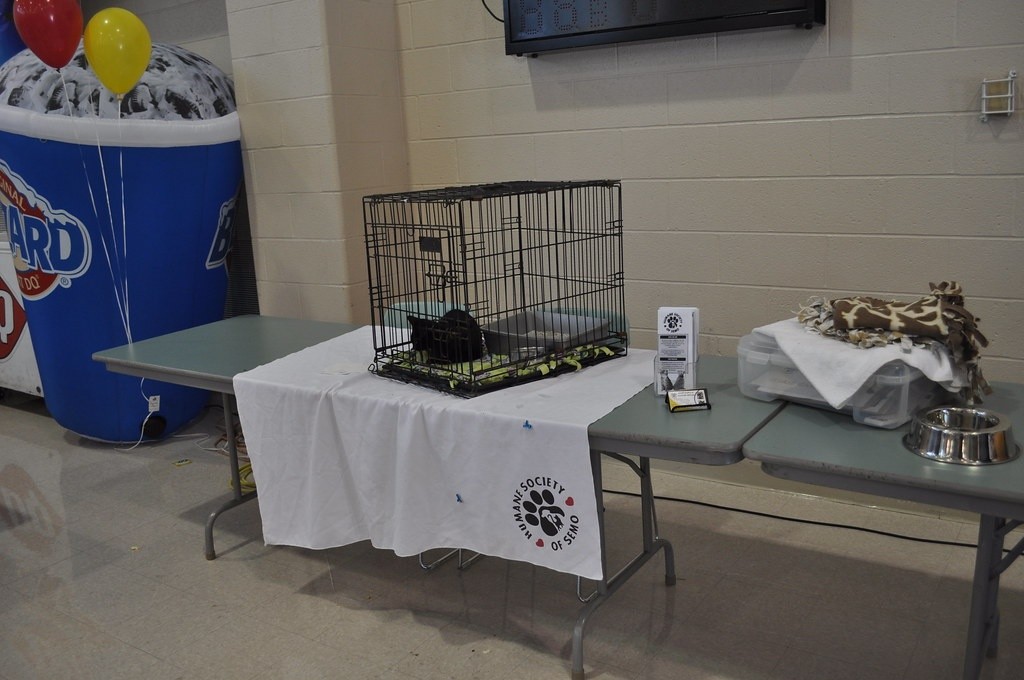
[85,6,152,100]
[12,0,84,73]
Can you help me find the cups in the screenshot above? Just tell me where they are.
[0,42,241,444]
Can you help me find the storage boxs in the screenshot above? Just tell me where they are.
[481,310,612,358]
[737,332,954,429]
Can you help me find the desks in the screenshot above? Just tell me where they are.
[91,315,787,680]
[741,380,1024,680]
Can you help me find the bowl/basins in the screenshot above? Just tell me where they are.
[902,408,1020,466]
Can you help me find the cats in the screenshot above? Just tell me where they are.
[407,309,482,363]
